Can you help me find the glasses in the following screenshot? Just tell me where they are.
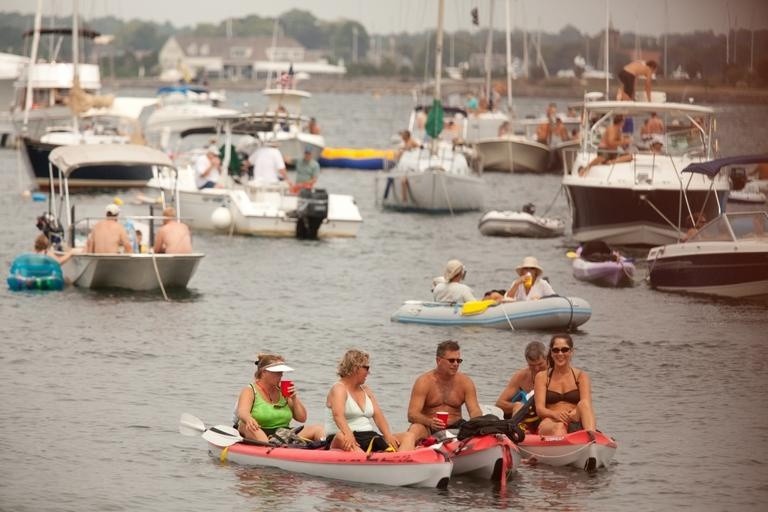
[356,365,369,371]
[269,386,288,408]
[438,355,463,363]
[552,347,571,353]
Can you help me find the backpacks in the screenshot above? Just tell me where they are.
[457,414,525,443]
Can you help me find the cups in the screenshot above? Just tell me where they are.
[281,380,291,396]
[436,411,450,429]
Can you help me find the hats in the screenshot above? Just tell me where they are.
[515,257,543,276]
[444,260,463,283]
[398,129,410,135]
[259,361,296,373]
[305,145,313,153]
[208,145,221,156]
[265,137,282,147]
[105,203,121,216]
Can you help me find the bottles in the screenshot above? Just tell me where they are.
[525,273,532,288]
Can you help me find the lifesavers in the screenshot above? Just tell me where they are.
[124,223,140,255]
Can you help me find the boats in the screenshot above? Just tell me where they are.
[390,295,593,333]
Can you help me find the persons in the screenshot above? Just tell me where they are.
[683,211,707,238]
[325,349,416,453]
[495,341,551,419]
[432,259,503,303]
[192,105,321,194]
[256,379,278,404]
[744,214,768,237]
[408,341,483,442]
[394,59,708,177]
[710,218,733,240]
[501,256,555,302]
[236,355,324,443]
[749,162,768,179]
[33,204,195,266]
[534,334,596,435]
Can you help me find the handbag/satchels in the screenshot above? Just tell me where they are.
[268,427,307,448]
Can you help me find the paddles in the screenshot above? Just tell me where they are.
[402,299,465,310]
[203,424,310,449]
[179,412,209,432]
